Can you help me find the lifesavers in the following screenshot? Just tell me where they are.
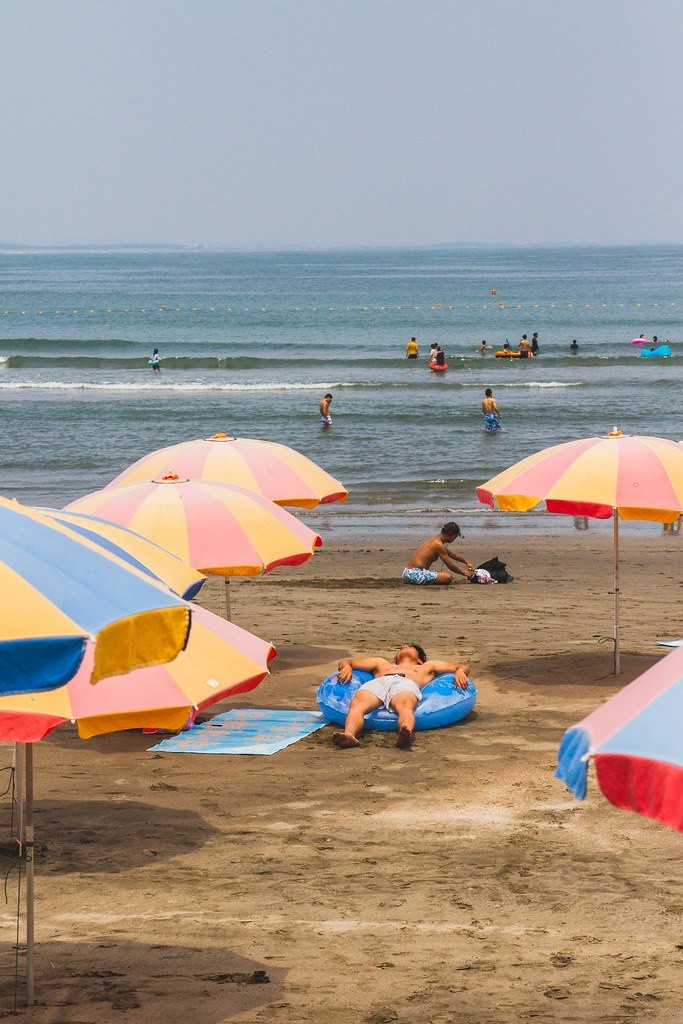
[429,363,447,371]
[632,338,646,344]
[316,660,478,731]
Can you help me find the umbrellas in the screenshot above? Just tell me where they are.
[553,646,683,834]
[0,601,277,854]
[475,426,683,677]
[11,497,207,601]
[103,433,349,622]
[60,469,323,576]
[1,495,193,1006]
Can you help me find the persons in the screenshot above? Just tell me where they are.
[640,334,657,342]
[483,389,502,432]
[401,522,475,585]
[570,340,578,348]
[406,337,419,359]
[477,341,486,352]
[427,343,444,371]
[332,643,471,750]
[504,333,539,359]
[151,349,161,372]
[664,516,682,535]
[319,394,333,430]
[665,340,668,342]
